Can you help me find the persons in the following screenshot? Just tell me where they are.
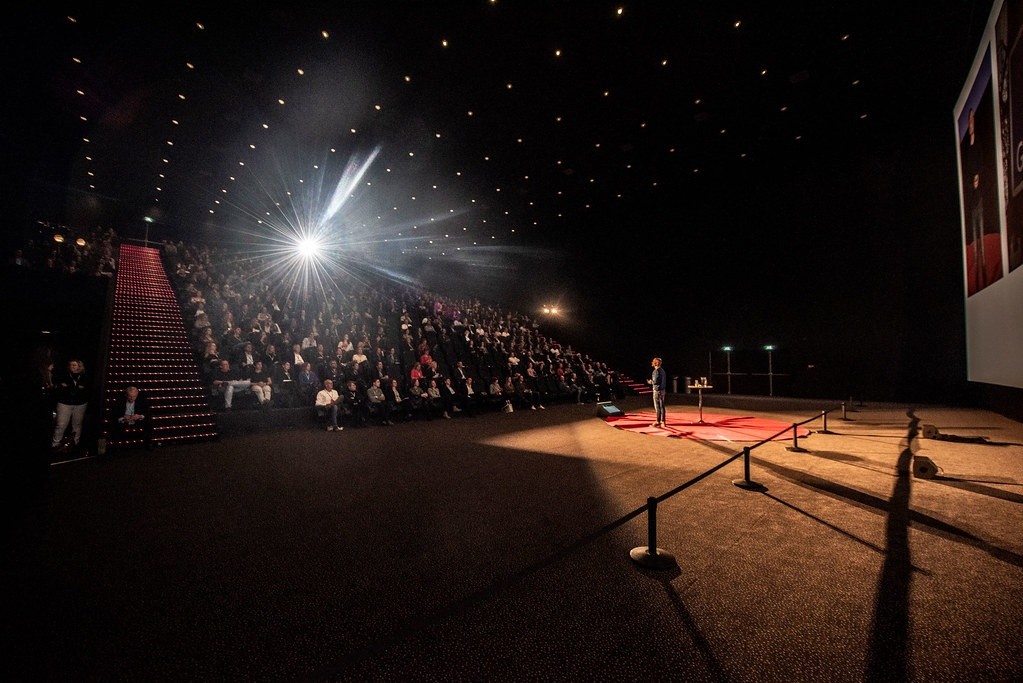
[965,107,987,291]
[112,387,153,451]
[159,239,624,430]
[0,221,116,459]
[649,358,665,427]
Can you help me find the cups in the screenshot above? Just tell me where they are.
[700,377,707,386]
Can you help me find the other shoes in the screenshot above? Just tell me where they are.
[222,407,231,412]
[334,426,341,431]
[531,405,536,410]
[325,425,333,431]
[453,406,462,412]
[537,404,545,409]
[387,420,394,425]
[649,421,659,427]
[381,421,386,425]
[145,441,154,451]
[577,401,584,405]
[661,422,665,427]
[443,413,451,419]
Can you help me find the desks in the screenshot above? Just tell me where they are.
[688,385,714,426]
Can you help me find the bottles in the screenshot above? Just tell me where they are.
[695,380,699,387]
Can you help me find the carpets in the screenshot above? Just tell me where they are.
[602,411,812,443]
